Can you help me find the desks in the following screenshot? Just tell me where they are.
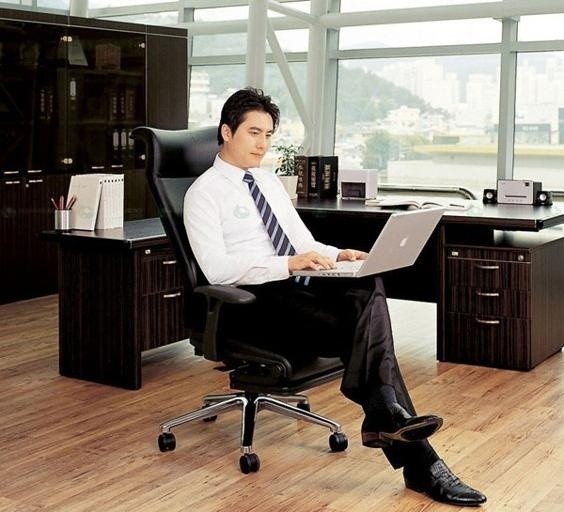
[35,186,564,393]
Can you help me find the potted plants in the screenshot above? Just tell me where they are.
[269,143,308,201]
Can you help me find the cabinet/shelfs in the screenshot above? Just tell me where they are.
[1,2,192,308]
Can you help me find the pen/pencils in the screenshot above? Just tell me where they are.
[450,204,464,207]
[51,195,77,210]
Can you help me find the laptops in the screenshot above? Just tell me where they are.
[292,207,447,278]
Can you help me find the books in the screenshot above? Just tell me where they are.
[96,86,137,122]
[95,43,121,70]
[66,173,125,231]
[35,90,55,119]
[105,127,136,170]
[379,196,473,211]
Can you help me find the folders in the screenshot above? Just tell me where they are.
[67,174,125,231]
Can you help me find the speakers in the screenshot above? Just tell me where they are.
[483,189,497,204]
[536,191,552,205]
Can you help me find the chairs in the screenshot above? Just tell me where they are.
[127,113,361,475]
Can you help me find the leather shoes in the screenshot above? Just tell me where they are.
[361,403,444,448]
[403,459,487,505]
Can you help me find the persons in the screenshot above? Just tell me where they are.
[182,85,487,507]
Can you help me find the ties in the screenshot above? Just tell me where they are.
[243,171,310,287]
